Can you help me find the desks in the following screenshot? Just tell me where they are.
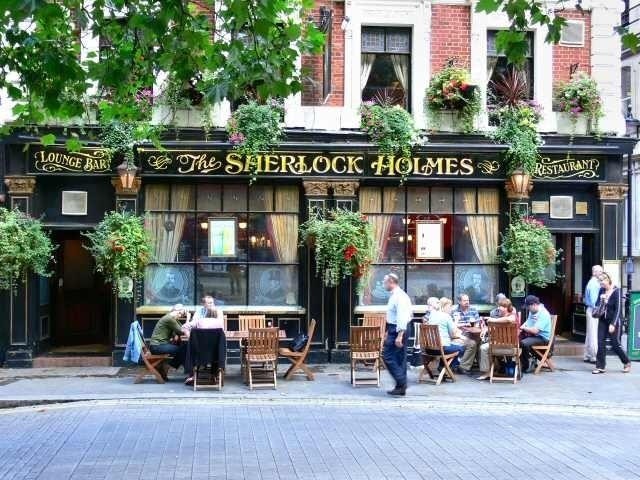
[461,326,495,340]
[178,329,287,341]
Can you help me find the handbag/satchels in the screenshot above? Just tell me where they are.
[592,307,607,318]
[288,333,309,352]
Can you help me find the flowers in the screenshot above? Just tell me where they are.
[296,205,376,288]
[225,96,289,187]
[359,98,429,188]
[557,70,607,166]
[424,66,482,136]
[91,84,165,163]
[485,99,545,176]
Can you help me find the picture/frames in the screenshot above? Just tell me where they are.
[415,220,444,259]
[207,216,238,258]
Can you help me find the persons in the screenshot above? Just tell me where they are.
[519,295,555,374]
[592,272,631,374]
[465,272,487,304]
[226,256,242,297]
[382,273,414,396]
[583,264,603,363]
[265,270,288,299]
[157,273,181,298]
[190,295,224,329]
[422,292,520,380]
[149,303,190,382]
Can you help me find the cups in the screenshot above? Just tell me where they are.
[470,318,474,327]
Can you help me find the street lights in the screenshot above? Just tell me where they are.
[625,104,640,331]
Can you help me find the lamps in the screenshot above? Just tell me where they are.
[116,161,138,189]
[510,164,530,194]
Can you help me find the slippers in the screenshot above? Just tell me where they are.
[592,369,607,374]
[623,360,631,373]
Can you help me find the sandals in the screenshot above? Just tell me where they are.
[476,371,491,379]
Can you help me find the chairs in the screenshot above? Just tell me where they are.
[244,326,280,391]
[531,314,558,375]
[238,314,268,376]
[278,318,316,381]
[134,336,174,384]
[418,323,460,385]
[487,321,522,384]
[193,364,224,391]
[349,312,387,388]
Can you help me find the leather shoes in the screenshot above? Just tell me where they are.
[458,367,471,375]
[584,357,596,364]
[387,383,407,395]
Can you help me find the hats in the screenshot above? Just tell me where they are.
[521,295,537,309]
